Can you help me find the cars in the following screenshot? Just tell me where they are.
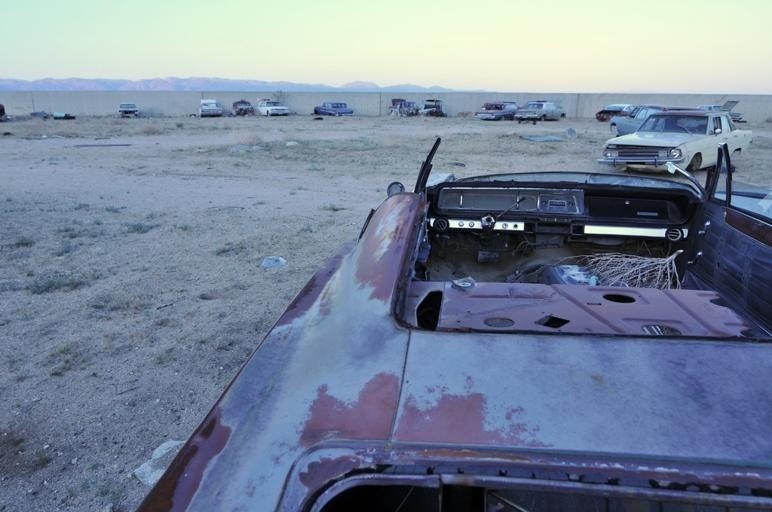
[596,110,754,172]
[472,100,561,122]
